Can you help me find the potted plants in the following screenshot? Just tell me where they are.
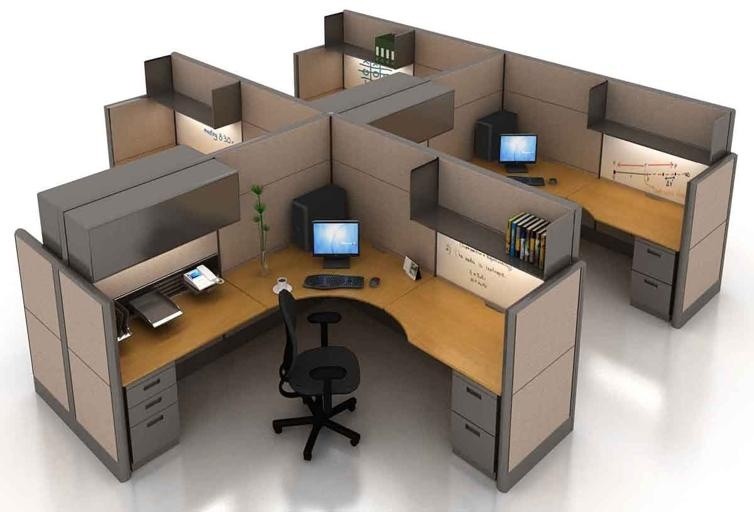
[250,182,272,276]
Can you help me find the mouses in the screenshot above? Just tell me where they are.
[550,178,558,184]
[369,276,380,287]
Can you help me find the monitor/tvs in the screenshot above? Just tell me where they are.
[312,219,361,269]
[499,133,539,173]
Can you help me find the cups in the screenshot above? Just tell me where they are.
[277,277,287,289]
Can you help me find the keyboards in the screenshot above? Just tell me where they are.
[303,274,364,290]
[507,176,545,186]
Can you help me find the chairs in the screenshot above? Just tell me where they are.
[272,289,361,461]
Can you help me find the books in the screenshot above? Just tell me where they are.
[504,213,550,270]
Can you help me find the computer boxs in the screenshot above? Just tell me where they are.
[473,110,518,162]
[291,183,349,251]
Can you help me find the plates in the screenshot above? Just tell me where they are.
[272,283,293,294]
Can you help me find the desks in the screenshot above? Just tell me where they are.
[466,154,685,322]
[118,238,504,481]
